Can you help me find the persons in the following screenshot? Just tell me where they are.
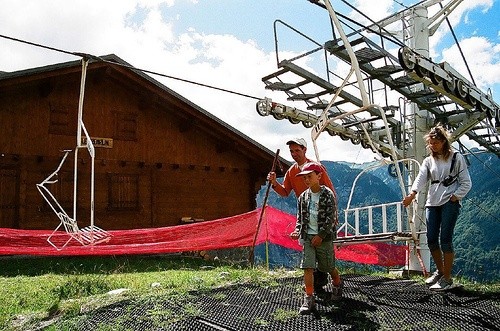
[402,126,473,291]
[290,163,345,315]
[266,138,337,291]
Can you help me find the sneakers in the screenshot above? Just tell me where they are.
[332,285,342,301]
[429,275,454,289]
[425,270,444,285]
[300,296,315,314]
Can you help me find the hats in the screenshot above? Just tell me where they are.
[286,137,307,149]
[295,162,322,177]
